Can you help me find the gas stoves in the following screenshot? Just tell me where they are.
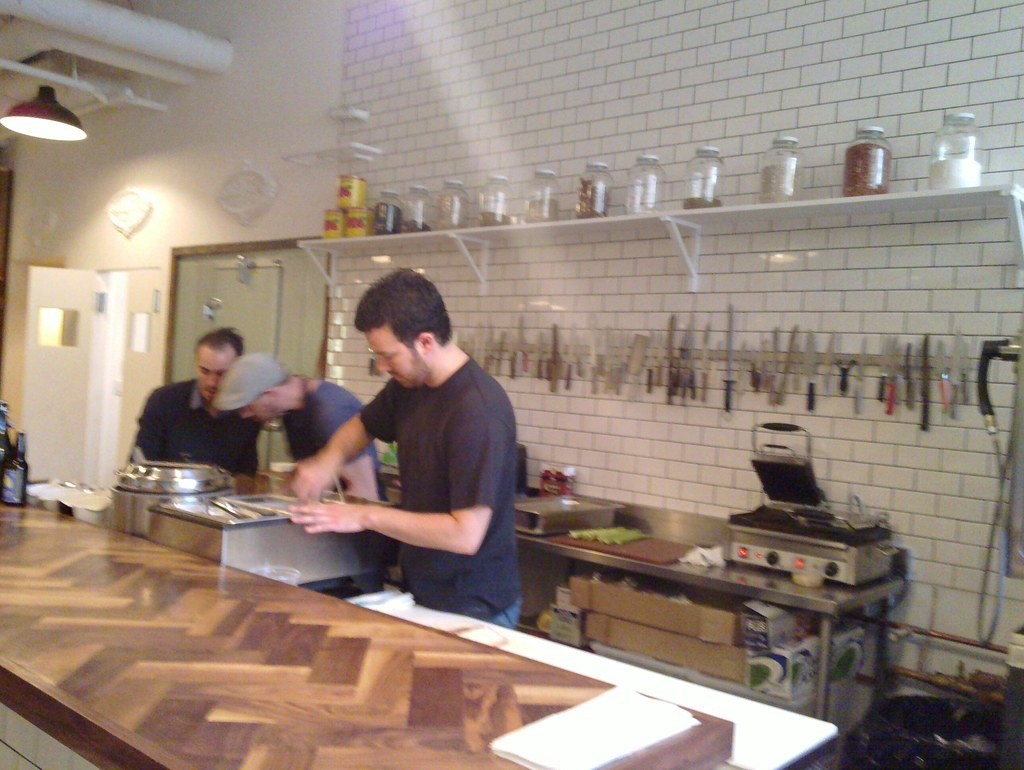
[728,505,892,585]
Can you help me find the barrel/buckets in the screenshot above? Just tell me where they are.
[109,459,237,542]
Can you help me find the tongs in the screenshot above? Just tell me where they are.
[209,497,291,521]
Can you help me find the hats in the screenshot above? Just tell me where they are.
[211,352,287,411]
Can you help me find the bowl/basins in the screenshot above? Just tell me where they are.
[790,564,826,588]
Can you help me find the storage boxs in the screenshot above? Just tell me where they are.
[549,602,582,644]
[747,625,865,699]
[568,571,739,646]
[744,601,816,646]
[555,584,571,603]
[584,611,743,681]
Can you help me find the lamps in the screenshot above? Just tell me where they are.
[106,189,154,237]
[0,41,87,141]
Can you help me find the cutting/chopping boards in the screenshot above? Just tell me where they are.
[549,535,691,563]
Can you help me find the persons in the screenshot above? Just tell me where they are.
[213,348,397,591]
[128,328,258,492]
[288,267,522,628]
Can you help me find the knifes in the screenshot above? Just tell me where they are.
[366,303,972,431]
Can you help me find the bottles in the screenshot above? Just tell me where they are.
[758,136,805,204]
[929,112,985,189]
[574,160,615,219]
[623,153,669,216]
[682,145,727,210]
[0,401,28,507]
[522,169,561,222]
[371,174,471,236]
[477,174,512,227]
[841,125,893,197]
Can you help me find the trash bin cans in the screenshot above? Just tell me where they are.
[868,695,1002,770]
